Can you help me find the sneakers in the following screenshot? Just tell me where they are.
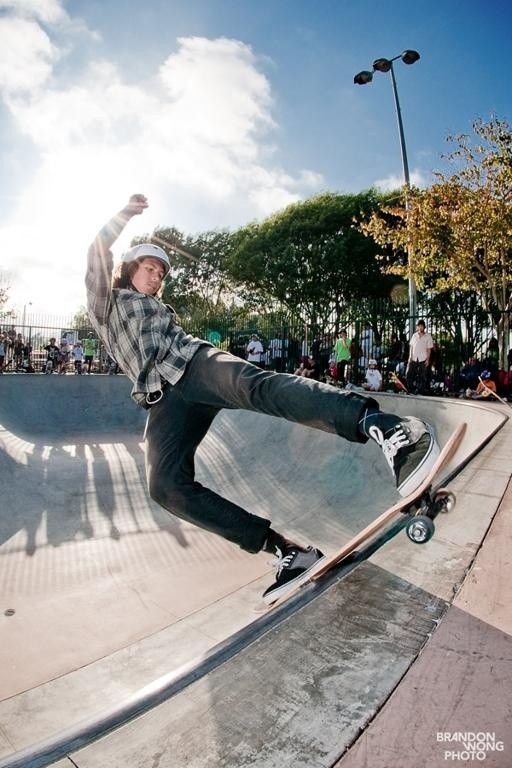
[261,545,327,605]
[368,415,440,498]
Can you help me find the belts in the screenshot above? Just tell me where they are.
[138,381,177,411]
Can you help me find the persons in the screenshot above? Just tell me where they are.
[0,330,98,375]
[228,320,512,401]
[85,194,441,606]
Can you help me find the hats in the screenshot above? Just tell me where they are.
[340,329,347,334]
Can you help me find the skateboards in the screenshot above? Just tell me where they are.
[389,371,415,396]
[478,376,512,409]
[252,421,467,614]
[45,360,53,375]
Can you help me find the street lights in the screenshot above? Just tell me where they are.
[22,301,33,345]
[352,48,421,340]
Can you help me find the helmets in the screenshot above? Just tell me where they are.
[121,244,171,281]
[328,358,335,364]
[48,331,94,345]
[480,370,491,380]
[368,359,377,365]
